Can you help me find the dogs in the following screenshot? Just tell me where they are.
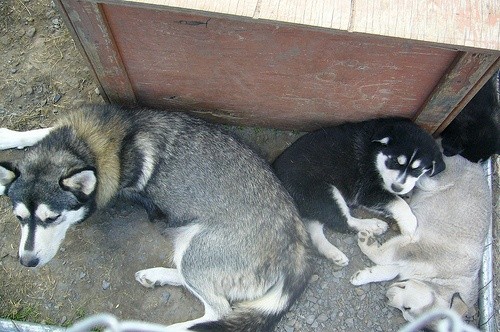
[273,116,446,267]
[351,154,491,323]
[0,103,315,332]
